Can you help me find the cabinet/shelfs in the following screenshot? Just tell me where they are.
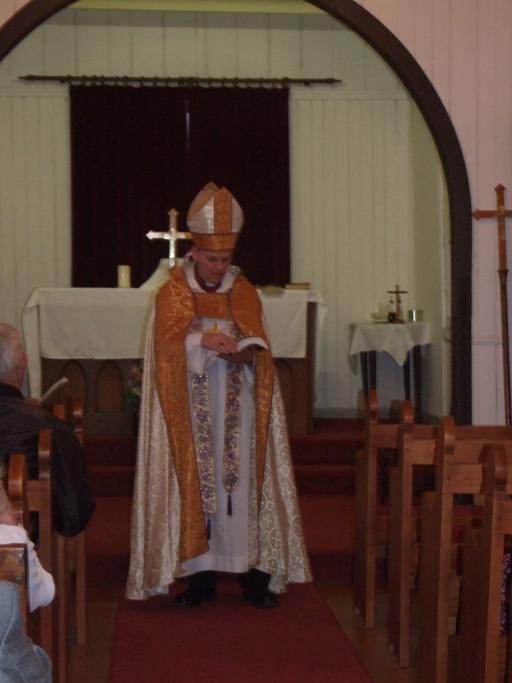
[32,284,318,436]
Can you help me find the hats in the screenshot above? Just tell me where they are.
[187,182,244,251]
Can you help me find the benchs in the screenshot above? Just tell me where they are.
[352,392,512,683]
[0,400,91,683]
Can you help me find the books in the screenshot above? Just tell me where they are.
[186,336,269,376]
[283,281,309,289]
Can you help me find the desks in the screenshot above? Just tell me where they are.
[353,323,429,422]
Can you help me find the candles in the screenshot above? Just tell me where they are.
[117,264,135,288]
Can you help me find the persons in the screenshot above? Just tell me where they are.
[1,320,97,548]
[1,579,56,682]
[2,478,56,613]
[123,246,313,609]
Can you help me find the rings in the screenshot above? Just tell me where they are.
[219,342,224,349]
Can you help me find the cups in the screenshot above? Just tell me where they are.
[409,310,423,322]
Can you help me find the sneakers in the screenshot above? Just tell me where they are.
[173,582,215,609]
[240,584,278,607]
